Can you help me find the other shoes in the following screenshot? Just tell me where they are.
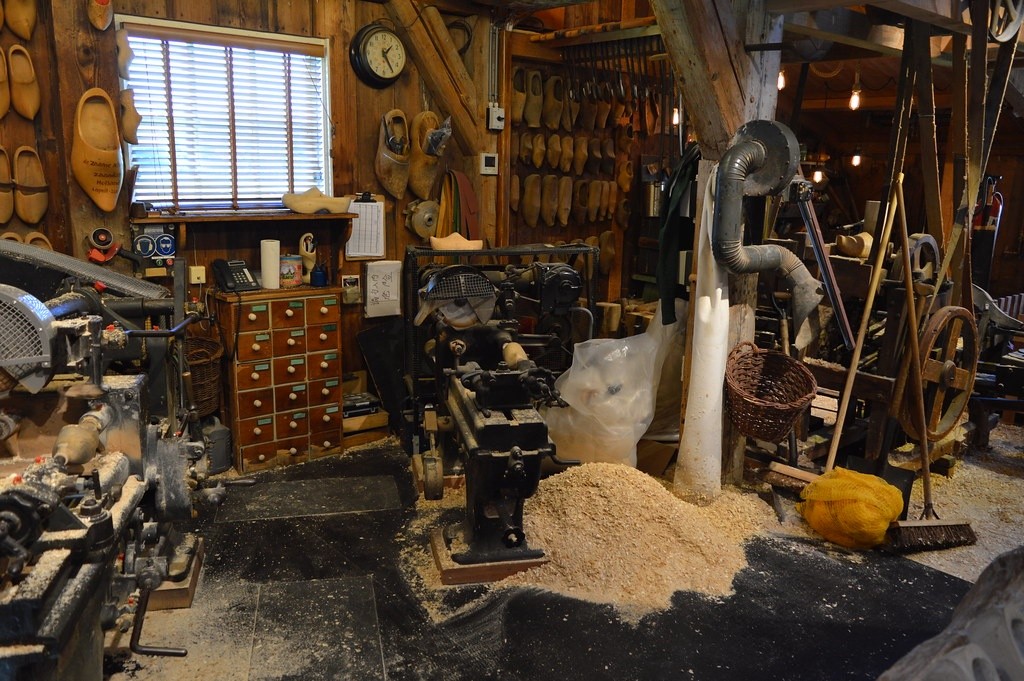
[508,65,660,283]
[116,29,134,81]
[375,110,446,200]
[0,146,49,225]
[0,0,38,40]
[0,44,41,121]
[119,89,142,145]
[0,231,54,251]
[71,87,124,212]
[88,0,113,30]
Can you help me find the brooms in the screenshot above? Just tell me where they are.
[890,175,978,552]
[754,171,907,496]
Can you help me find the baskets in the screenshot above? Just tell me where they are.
[725,341,817,444]
[183,326,225,420]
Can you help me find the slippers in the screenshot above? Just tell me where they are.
[282,186,351,214]
[430,232,484,250]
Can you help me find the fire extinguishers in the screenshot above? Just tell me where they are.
[954,174,1004,256]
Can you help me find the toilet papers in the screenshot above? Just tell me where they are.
[260,240,280,289]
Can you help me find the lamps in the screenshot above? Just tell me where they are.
[778,64,786,90]
[851,147,861,167]
[812,163,822,183]
[673,103,679,126]
[848,71,862,110]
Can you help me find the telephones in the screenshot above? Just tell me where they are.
[211,259,261,294]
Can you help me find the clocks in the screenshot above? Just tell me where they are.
[348,19,407,90]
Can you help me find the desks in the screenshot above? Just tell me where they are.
[128,212,358,272]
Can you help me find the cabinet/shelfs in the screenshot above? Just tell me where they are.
[401,242,600,455]
[202,284,347,476]
